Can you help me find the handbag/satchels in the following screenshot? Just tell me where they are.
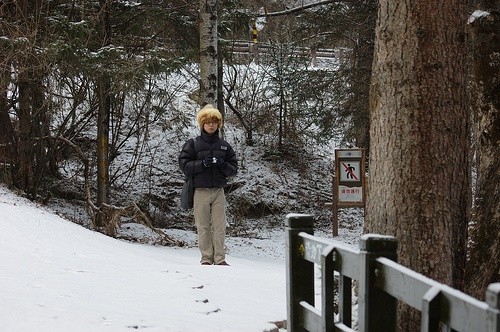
[181,178,193,209]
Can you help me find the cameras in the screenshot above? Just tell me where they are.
[211,157,217,163]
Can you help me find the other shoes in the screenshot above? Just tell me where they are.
[201,262,211,265]
[214,261,229,265]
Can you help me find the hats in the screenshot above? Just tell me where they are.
[196,104,222,128]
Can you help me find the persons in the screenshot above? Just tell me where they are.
[178,104,238,265]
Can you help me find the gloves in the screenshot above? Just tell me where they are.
[217,158,224,167]
[205,155,215,167]
